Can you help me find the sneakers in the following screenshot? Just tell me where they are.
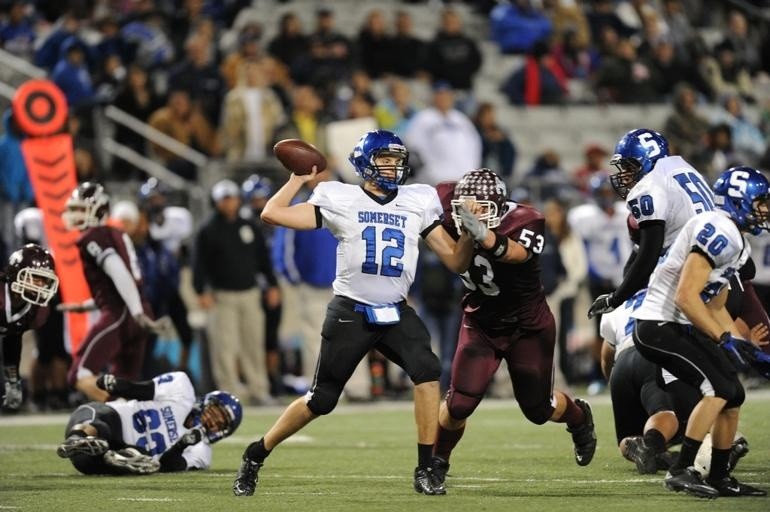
[104,450,161,474]
[57,433,110,459]
[234,442,262,497]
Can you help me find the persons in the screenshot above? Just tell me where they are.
[432,166,601,479]
[58,369,243,479]
[0,0,769,415]
[230,130,484,498]
[631,166,770,501]
[587,128,756,485]
[600,253,682,477]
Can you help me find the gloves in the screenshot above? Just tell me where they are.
[4,380,23,409]
[718,331,770,374]
[456,205,487,243]
[587,292,621,319]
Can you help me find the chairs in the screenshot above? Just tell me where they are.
[487,96,674,184]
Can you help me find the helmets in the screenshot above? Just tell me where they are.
[63,181,112,229]
[6,243,58,308]
[348,130,411,193]
[191,390,242,445]
[450,168,506,235]
[712,166,770,236]
[609,128,669,200]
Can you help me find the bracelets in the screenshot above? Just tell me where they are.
[487,232,508,260]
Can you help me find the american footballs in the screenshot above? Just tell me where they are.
[274,139,326,175]
